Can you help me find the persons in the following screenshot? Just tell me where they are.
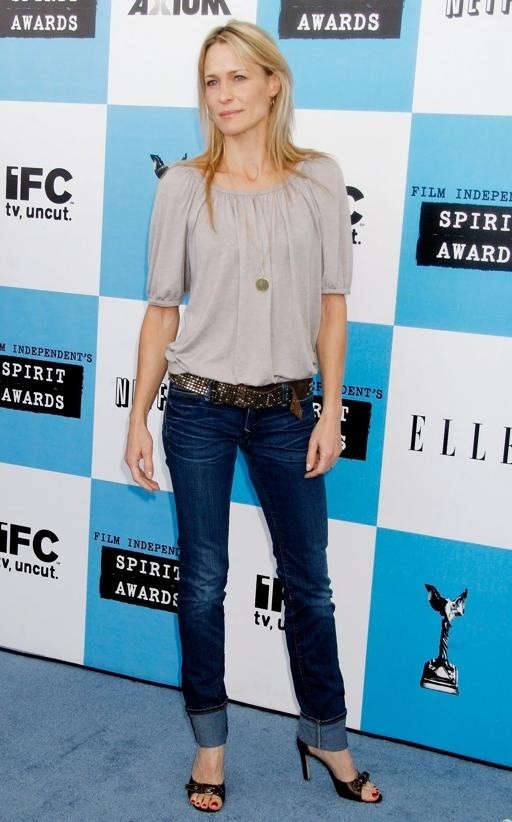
[123,20,382,813]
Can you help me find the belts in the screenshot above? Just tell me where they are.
[168,373,314,421]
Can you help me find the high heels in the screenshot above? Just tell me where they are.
[184,775,225,812]
[297,736,382,803]
